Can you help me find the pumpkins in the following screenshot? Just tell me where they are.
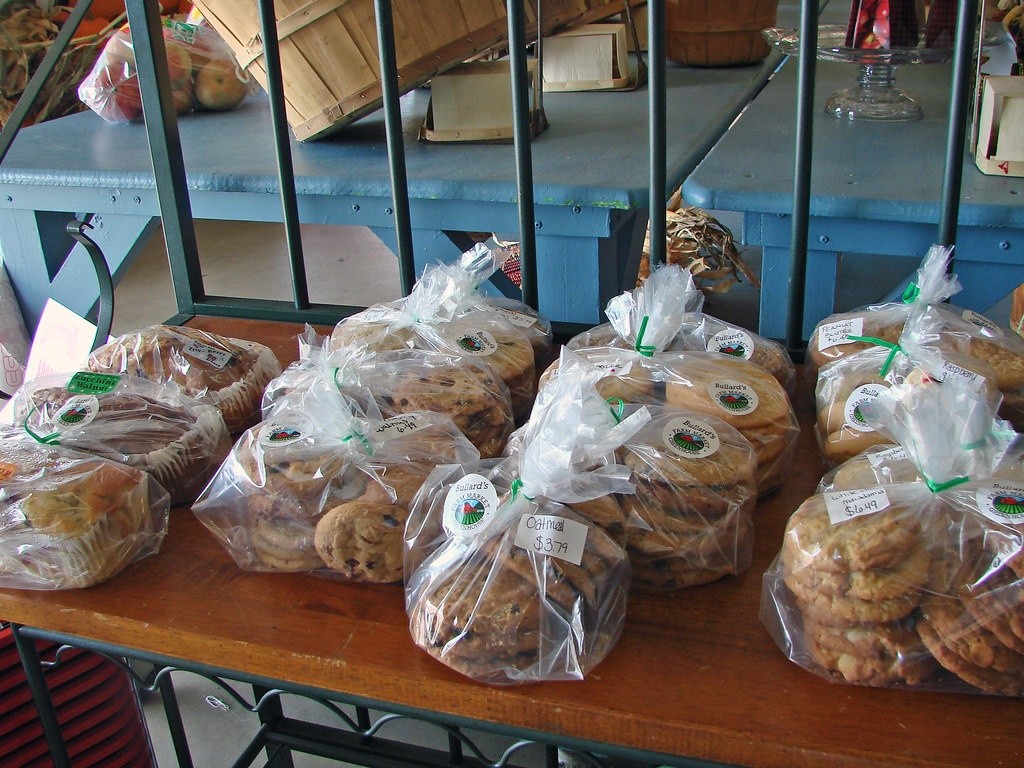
[44,0,193,54]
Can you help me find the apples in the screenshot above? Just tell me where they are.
[83,15,247,123]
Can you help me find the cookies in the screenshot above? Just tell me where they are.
[237,299,798,685]
[778,313,1024,700]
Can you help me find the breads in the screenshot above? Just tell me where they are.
[0,323,282,590]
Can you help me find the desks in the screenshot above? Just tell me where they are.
[0,313,1024,768]
[0,0,1024,346]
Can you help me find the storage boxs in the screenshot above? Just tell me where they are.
[532,23,635,93]
[421,58,545,140]
[976,75,1024,179]
